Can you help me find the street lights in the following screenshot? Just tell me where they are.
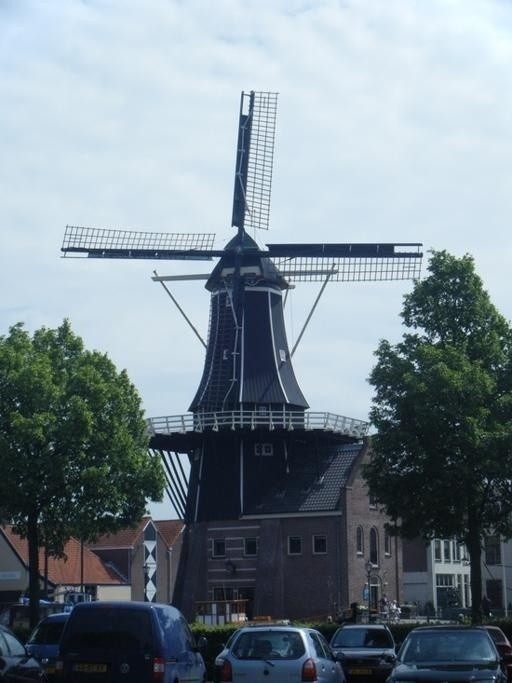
[350,549,373,614]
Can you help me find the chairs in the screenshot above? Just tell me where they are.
[249,640,272,659]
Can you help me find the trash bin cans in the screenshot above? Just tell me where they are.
[400,604,416,619]
[354,605,370,625]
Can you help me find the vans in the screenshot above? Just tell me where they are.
[55,599,210,682]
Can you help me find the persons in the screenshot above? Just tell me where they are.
[389,599,402,619]
[378,592,390,618]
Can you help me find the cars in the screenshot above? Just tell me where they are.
[1,621,50,682]
[475,624,512,667]
[214,620,346,682]
[24,611,73,673]
[385,624,509,683]
[323,623,398,676]
